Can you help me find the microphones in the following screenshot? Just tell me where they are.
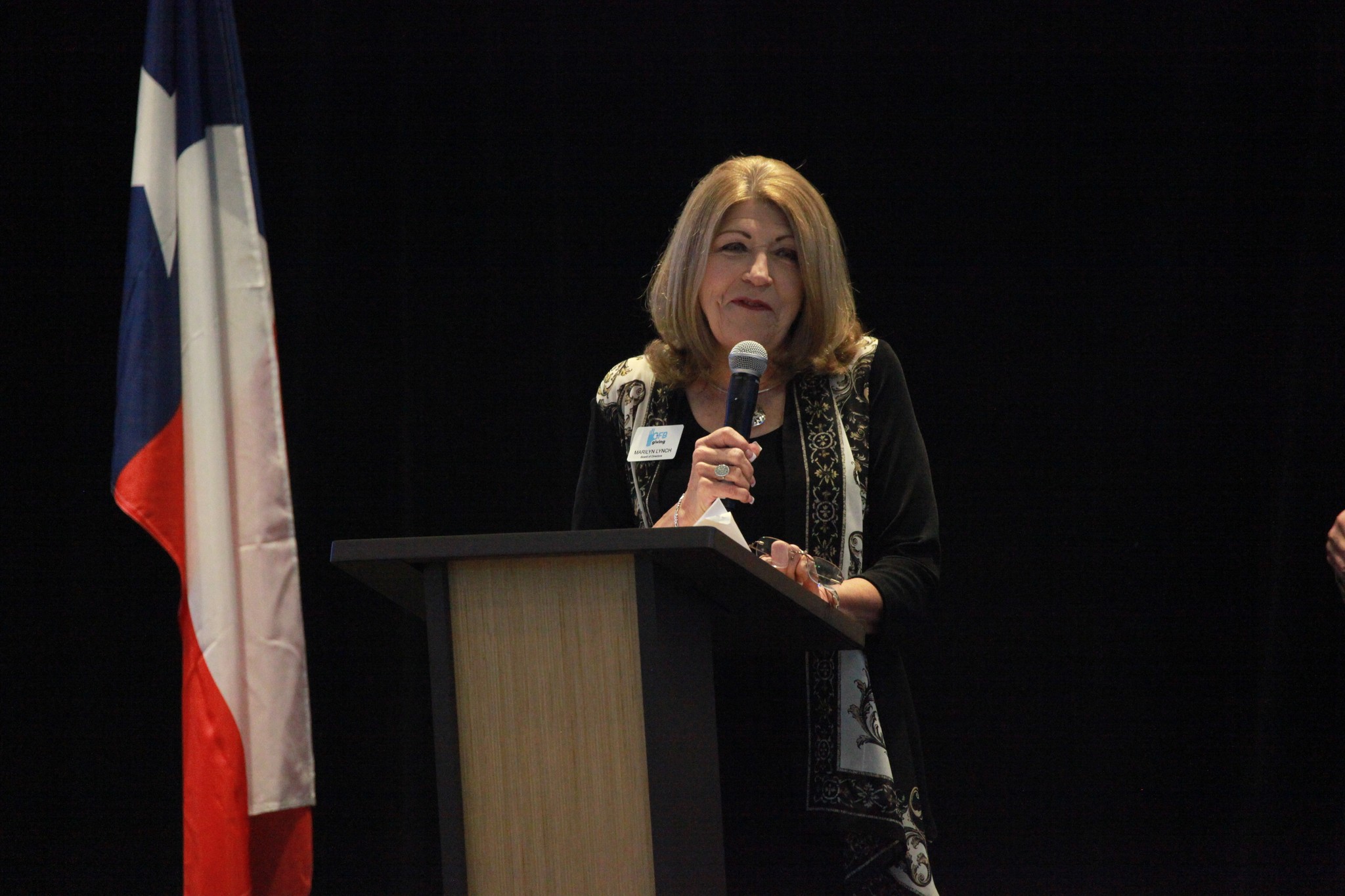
[724,339,768,441]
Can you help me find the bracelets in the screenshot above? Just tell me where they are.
[821,584,841,609]
[673,492,686,528]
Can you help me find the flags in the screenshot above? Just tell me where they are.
[103,1,325,896]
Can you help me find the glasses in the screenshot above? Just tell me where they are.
[747,536,845,591]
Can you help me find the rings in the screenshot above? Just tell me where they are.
[713,463,730,480]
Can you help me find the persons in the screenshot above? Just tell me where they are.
[1325,506,1345,600]
[568,152,944,896]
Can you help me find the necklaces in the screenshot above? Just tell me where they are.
[703,367,787,430]
